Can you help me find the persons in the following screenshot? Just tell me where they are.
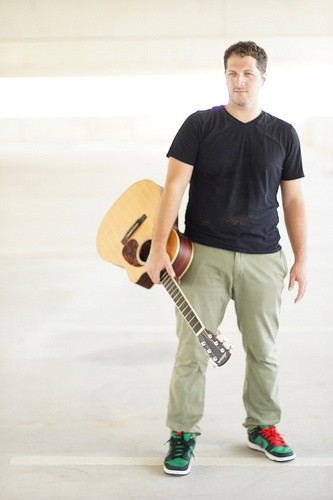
[145,41,308,476]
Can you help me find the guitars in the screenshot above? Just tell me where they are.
[95,179,234,368]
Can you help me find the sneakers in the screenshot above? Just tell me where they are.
[163,431,196,476]
[247,424,295,462]
